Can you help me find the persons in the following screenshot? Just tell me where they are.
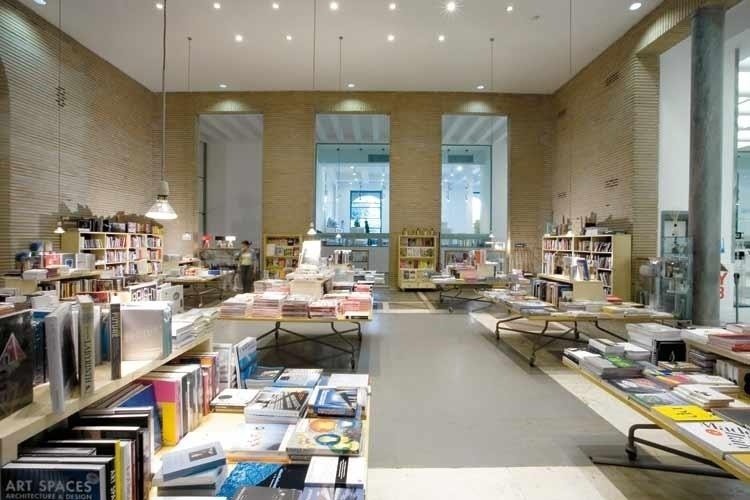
[233,239,257,293]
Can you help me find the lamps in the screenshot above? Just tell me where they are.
[306,0,317,235]
[144,0,180,221]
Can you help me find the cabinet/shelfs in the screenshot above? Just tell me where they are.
[397,235,438,292]
[263,234,303,281]
[538,232,632,301]
[61,231,164,292]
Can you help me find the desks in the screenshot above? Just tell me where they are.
[1,268,387,499]
[431,266,750,490]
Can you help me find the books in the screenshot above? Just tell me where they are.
[253,251,378,287]
[30,215,164,287]
[4,272,21,277]
[7,269,22,273]
[562,322,750,476]
[435,211,676,321]
[0,287,374,500]
